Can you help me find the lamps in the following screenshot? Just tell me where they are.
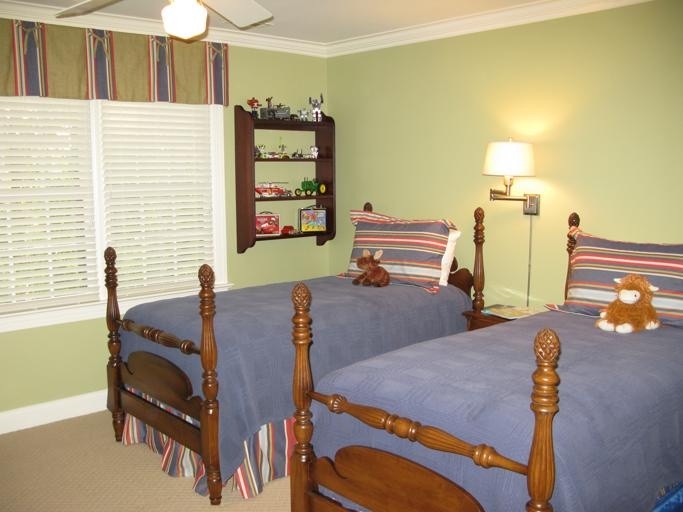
[157,1,211,44]
[478,136,540,217]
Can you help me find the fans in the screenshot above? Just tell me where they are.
[53,1,276,35]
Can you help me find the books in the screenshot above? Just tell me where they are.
[481,307,551,320]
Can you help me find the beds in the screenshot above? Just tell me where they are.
[100,199,487,509]
[285,211,683,512]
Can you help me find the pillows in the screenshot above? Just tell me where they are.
[436,230,460,287]
[546,221,681,330]
[337,208,460,298]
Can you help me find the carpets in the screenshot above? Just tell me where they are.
[651,478,683,511]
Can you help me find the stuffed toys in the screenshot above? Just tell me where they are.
[594,274,661,334]
[352,249,390,287]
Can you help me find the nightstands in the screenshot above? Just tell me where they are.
[459,302,538,330]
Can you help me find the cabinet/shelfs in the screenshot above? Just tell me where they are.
[233,100,338,254]
